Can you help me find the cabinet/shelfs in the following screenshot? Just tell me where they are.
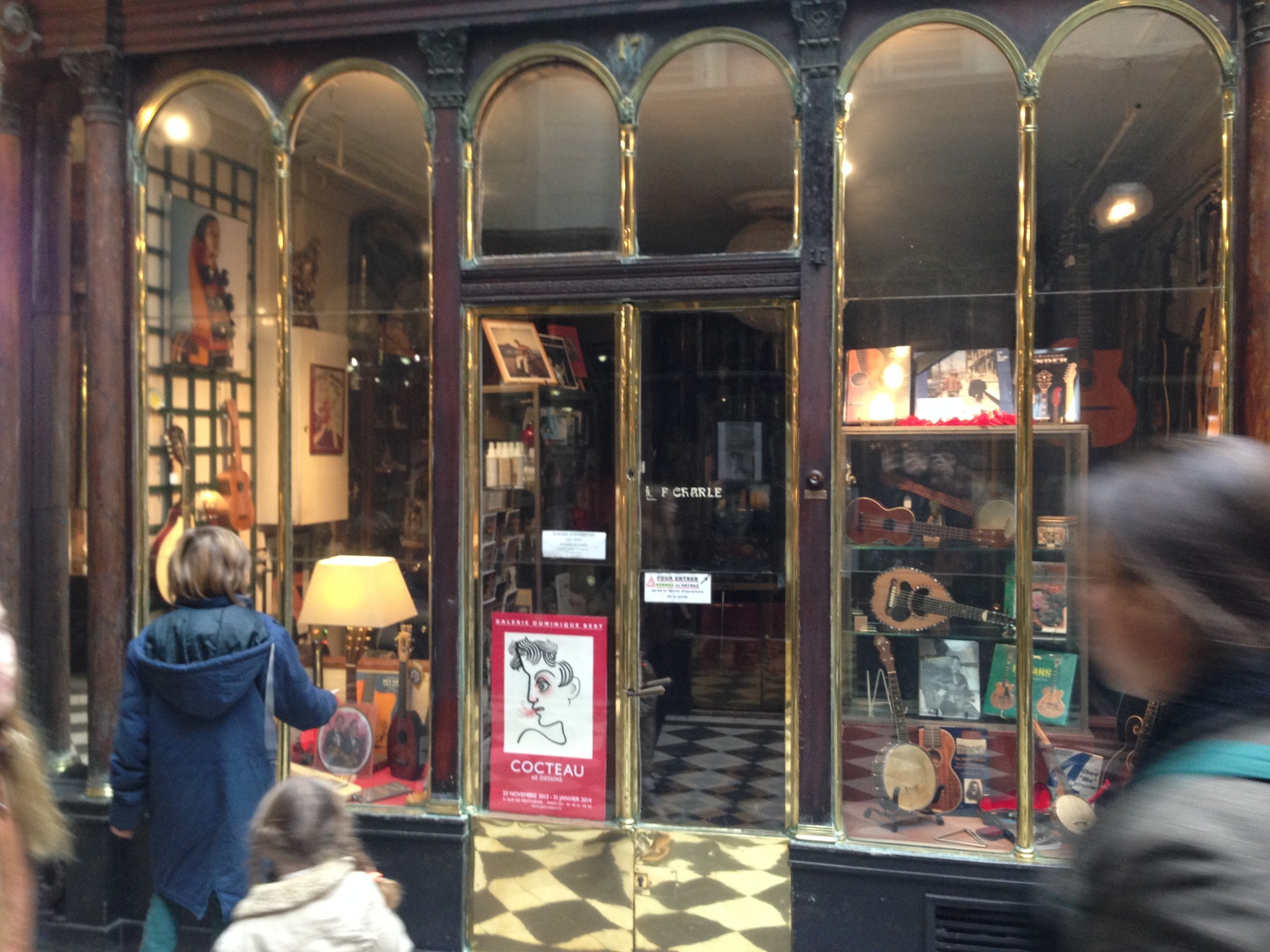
[837,422,1093,740]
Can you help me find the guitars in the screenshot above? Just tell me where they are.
[385,630,429,783]
[289,623,331,768]
[212,399,257,531]
[1032,717,1097,843]
[917,724,964,815]
[1098,699,1160,808]
[845,495,1008,550]
[312,624,379,779]
[148,425,199,611]
[868,565,1043,634]
[876,466,1017,544]
[1038,184,1139,450]
[1142,217,1179,447]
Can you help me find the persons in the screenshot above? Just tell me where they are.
[0,526,416,952]
[1066,431,1270,952]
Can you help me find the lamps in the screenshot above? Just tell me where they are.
[296,553,419,708]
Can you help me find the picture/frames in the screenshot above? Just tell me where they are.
[481,316,559,385]
[915,636,983,721]
[307,362,348,458]
